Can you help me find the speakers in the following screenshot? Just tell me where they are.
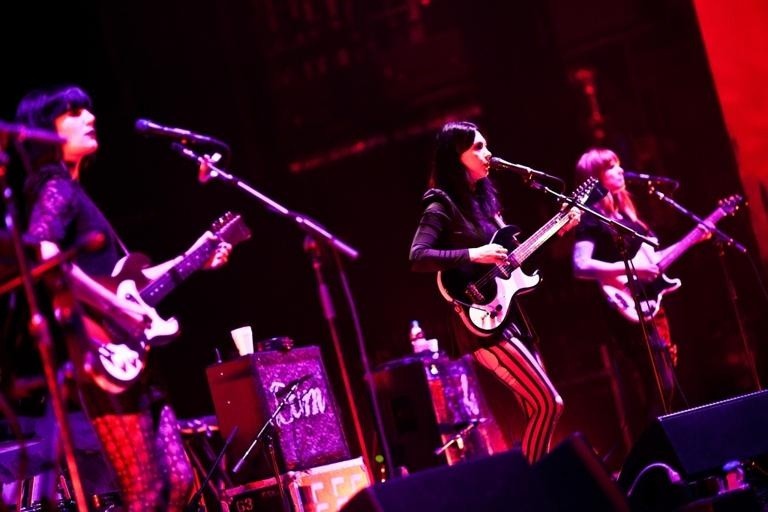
[204,346,352,484]
[365,350,494,476]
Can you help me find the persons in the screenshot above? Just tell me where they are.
[409,122,585,464]
[13,87,233,512]
[574,151,713,427]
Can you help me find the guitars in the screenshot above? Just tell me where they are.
[63,209,254,395]
[434,176,600,336]
[602,193,743,324]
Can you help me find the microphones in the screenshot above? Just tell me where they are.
[622,172,681,191]
[273,373,313,398]
[0,124,67,148]
[135,119,231,155]
[488,157,563,184]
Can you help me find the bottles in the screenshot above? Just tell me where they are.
[409,321,428,354]
